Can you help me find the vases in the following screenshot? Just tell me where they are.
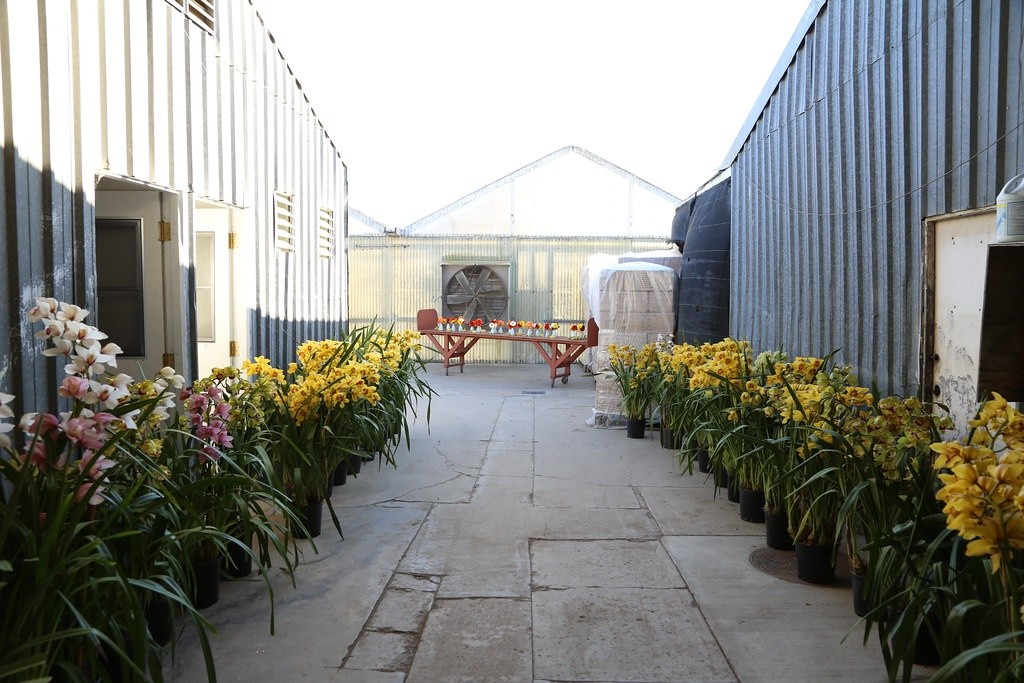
[137,420,403,644]
[438,323,584,340]
[627,419,944,683]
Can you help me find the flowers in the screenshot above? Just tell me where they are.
[0,294,438,683]
[437,318,585,331]
[611,332,1024,683]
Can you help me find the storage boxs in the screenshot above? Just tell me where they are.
[599,269,676,334]
